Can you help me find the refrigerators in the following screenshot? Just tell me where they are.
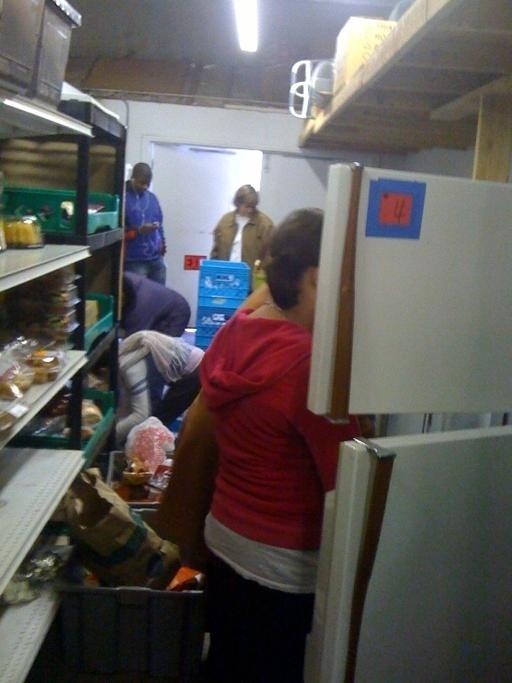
[306,162,511,682]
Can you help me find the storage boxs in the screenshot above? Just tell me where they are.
[1,0,82,105]
[47,505,213,682]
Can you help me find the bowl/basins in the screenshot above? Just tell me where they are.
[122,470,152,486]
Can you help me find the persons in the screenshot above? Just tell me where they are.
[83,161,379,683]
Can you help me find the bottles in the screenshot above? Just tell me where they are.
[129,456,151,472]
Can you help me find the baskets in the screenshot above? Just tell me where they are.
[195,258,252,347]
